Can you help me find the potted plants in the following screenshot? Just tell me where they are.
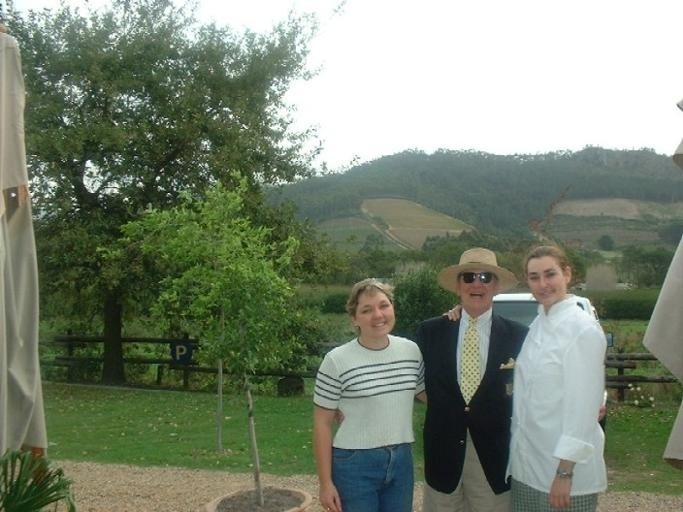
[88,170,311,512]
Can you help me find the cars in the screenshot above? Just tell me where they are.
[489,293,616,350]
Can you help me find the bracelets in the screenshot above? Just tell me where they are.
[555,470,574,479]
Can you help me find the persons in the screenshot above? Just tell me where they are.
[440,245,608,512]
[311,276,428,511]
[334,246,607,511]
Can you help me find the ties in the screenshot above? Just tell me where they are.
[461,318,481,406]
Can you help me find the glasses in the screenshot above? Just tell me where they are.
[463,272,491,283]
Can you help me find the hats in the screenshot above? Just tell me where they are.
[437,248,517,292]
